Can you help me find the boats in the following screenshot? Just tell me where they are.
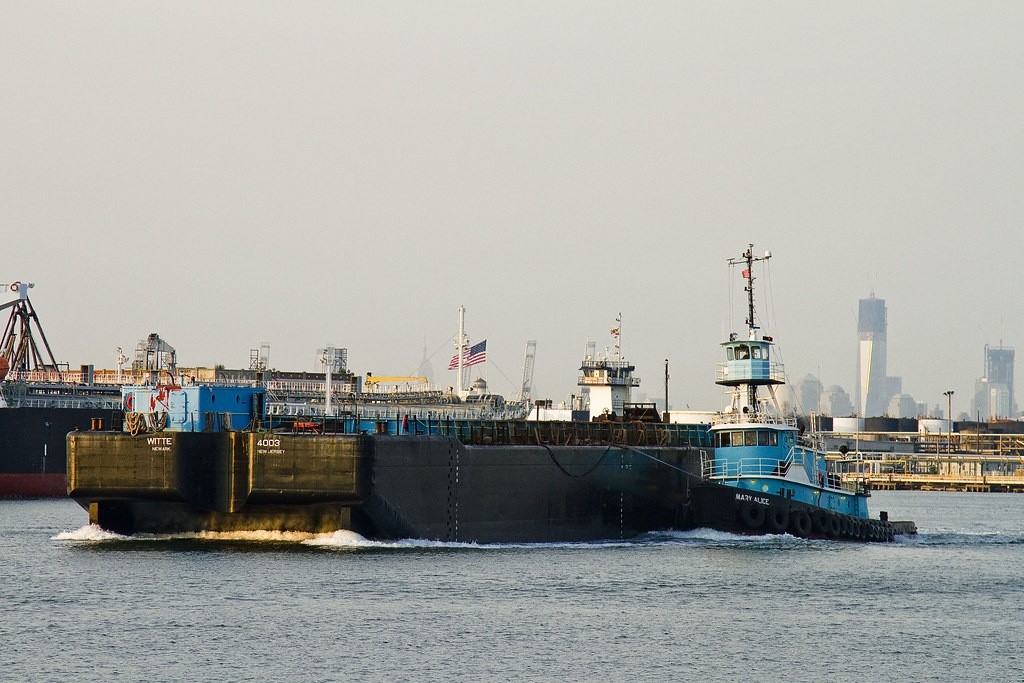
[63,239,922,544]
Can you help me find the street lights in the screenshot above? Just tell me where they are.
[943,391,956,474]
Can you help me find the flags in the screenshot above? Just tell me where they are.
[447,340,490,369]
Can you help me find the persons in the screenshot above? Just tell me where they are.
[818,470,824,487]
[401,412,409,435]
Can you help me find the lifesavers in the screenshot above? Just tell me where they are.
[127,396,135,411]
[10,283,18,292]
[742,500,895,541]
